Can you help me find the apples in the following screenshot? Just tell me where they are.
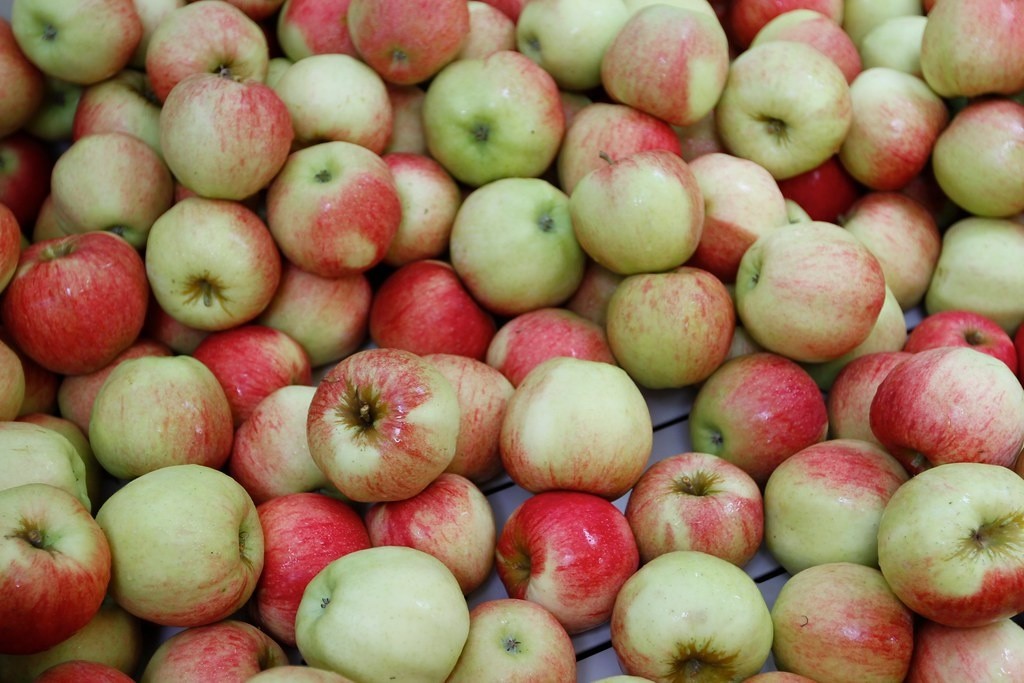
[0,0,1024,683]
